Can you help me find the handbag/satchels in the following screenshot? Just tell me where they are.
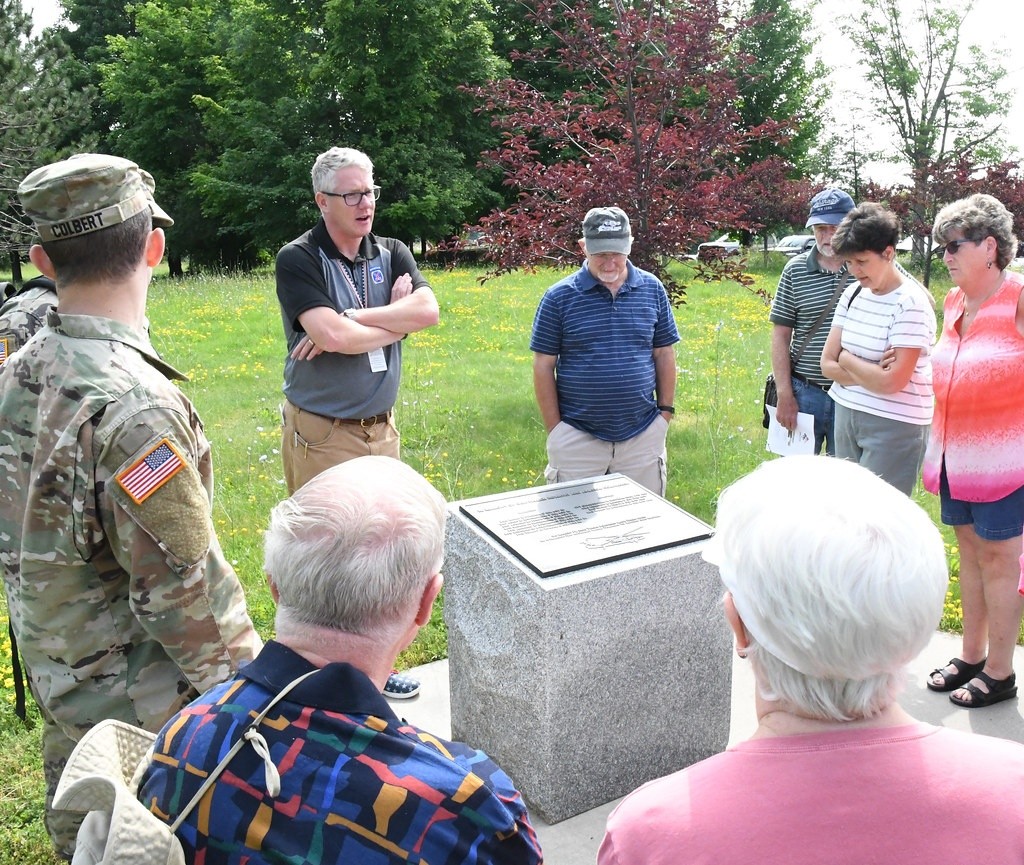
[763,374,777,429]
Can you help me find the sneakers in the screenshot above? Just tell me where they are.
[382,669,421,699]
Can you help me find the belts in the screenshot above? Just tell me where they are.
[300,408,391,428]
[790,369,831,393]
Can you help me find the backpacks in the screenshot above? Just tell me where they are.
[0,273,57,308]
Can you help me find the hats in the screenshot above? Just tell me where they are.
[804,187,857,229]
[68,153,175,228]
[51,719,186,865]
[582,207,632,255]
[17,159,149,242]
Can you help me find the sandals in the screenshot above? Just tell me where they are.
[949,671,1017,708]
[927,658,986,692]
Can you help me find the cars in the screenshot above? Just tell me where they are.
[895,233,945,258]
[758,234,815,260]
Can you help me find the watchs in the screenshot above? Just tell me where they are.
[342,307,357,322]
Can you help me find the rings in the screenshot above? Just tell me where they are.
[308,339,314,345]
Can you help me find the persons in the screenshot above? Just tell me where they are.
[529,206,680,500]
[769,188,936,458]
[0,153,265,865]
[820,202,937,498]
[595,453,1024,865]
[275,146,440,699]
[130,455,546,865]
[921,194,1024,708]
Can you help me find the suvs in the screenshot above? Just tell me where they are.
[697,230,778,263]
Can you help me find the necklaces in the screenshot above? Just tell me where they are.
[963,291,998,317]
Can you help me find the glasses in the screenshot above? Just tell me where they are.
[320,185,381,206]
[934,238,974,259]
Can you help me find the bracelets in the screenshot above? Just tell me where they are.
[657,405,675,414]
